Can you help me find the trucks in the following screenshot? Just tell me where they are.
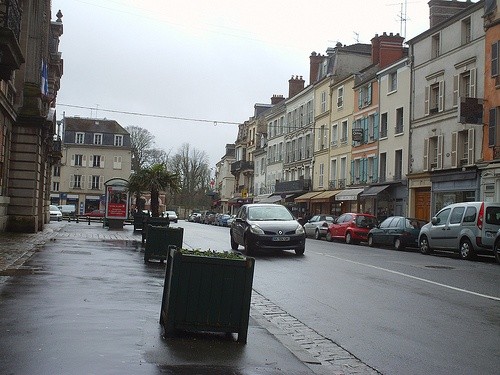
[57,205,75,216]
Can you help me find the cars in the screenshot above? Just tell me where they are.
[49,204,63,221]
[331,212,377,246]
[163,210,177,222]
[305,215,334,240]
[85,209,105,216]
[369,215,427,249]
[230,204,307,254]
[189,213,231,225]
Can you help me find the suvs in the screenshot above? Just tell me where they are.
[418,202,500,258]
